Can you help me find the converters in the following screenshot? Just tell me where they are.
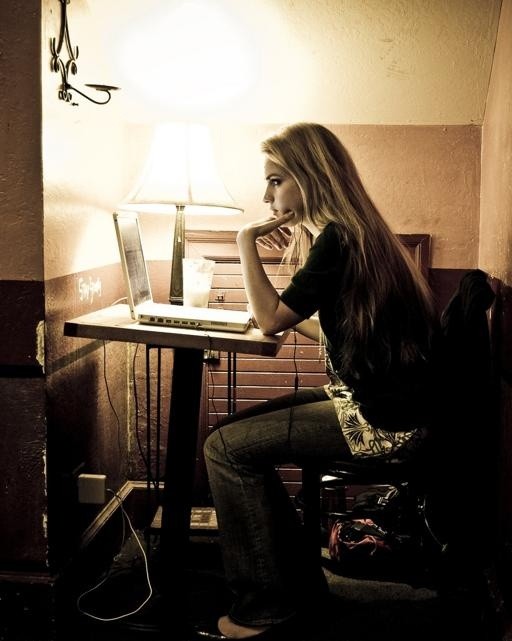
[78,474,107,504]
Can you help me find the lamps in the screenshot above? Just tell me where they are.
[119,122,243,305]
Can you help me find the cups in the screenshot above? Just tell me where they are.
[181,256,214,309]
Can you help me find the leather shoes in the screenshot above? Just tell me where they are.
[187,614,291,641]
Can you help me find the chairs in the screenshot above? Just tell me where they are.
[292,270,496,641]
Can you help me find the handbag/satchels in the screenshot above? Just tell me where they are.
[329,477,460,580]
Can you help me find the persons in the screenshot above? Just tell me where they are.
[188,123,453,641]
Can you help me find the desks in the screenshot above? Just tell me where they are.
[63,304,291,580]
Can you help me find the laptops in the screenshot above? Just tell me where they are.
[112,212,251,333]
